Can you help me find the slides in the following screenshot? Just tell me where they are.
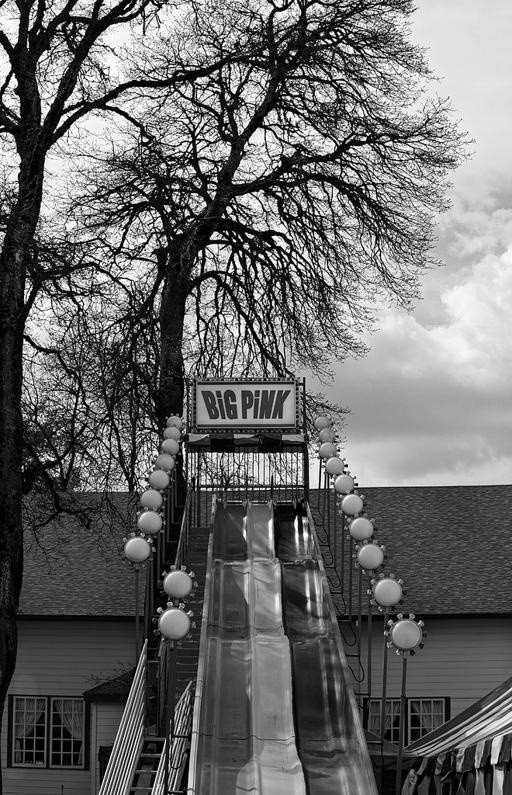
[186,495,381,795]
